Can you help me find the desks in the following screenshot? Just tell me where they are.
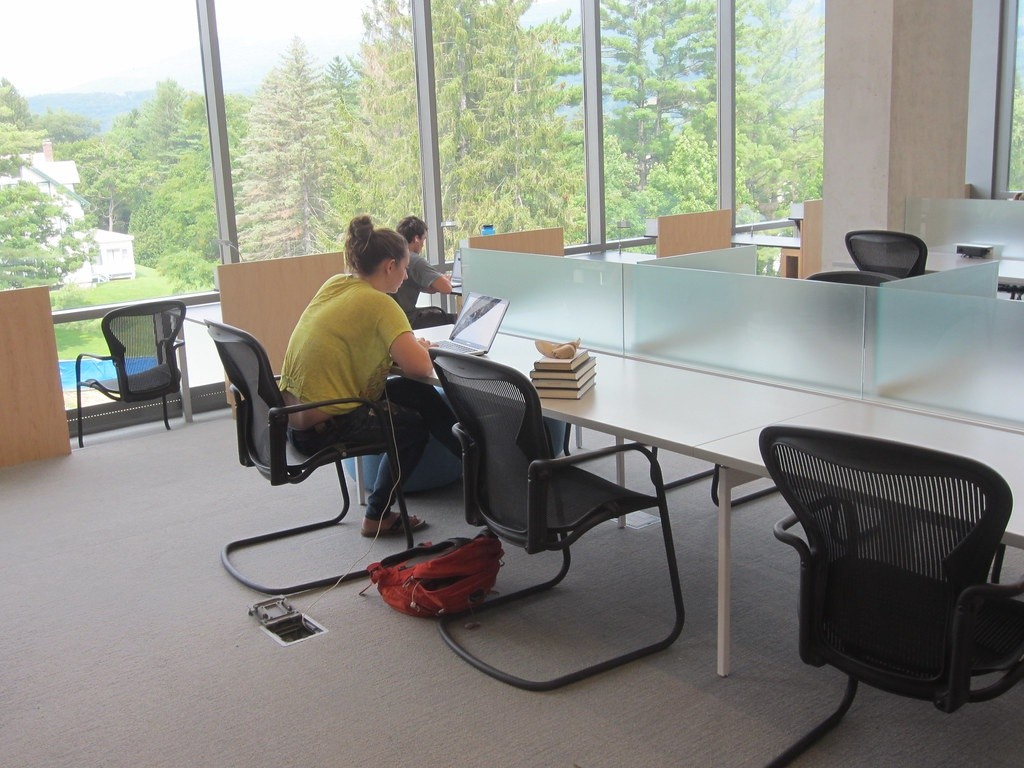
[566,250,657,263]
[730,234,801,248]
[413,323,1024,677]
[925,256,1024,300]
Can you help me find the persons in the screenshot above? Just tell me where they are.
[279,216,461,537]
[386,216,458,330]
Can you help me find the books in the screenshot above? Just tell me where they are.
[530,348,597,400]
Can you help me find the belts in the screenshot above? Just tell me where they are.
[292,414,345,444]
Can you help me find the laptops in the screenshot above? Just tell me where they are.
[428,293,510,357]
[450,249,462,288]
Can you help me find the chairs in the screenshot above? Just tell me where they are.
[756,423,1024,768]
[202,318,431,594]
[76,300,187,448]
[425,347,687,692]
[806,229,928,322]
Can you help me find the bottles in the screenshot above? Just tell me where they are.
[482,224,496,235]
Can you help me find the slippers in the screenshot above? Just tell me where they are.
[361,514,426,536]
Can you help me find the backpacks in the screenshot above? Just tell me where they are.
[367,528,504,618]
